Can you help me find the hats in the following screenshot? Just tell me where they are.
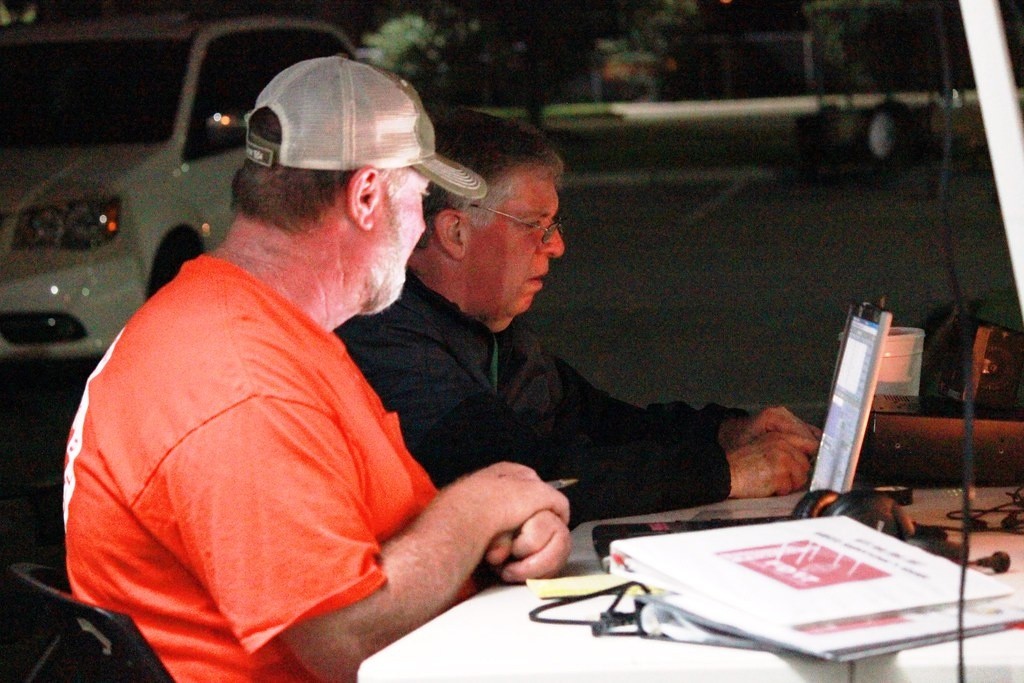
[245,55,487,200]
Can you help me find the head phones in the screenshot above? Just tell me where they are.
[796,487,968,563]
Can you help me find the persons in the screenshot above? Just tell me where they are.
[334,109,823,532]
[63,53,572,683]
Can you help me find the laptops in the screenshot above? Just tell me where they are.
[594,302,892,558]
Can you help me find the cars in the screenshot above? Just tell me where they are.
[0,6,363,364]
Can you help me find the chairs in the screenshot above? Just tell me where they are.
[8,560,177,683]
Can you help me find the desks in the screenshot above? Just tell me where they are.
[521,479,1024,578]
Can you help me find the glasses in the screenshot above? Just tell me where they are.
[431,200,565,243]
